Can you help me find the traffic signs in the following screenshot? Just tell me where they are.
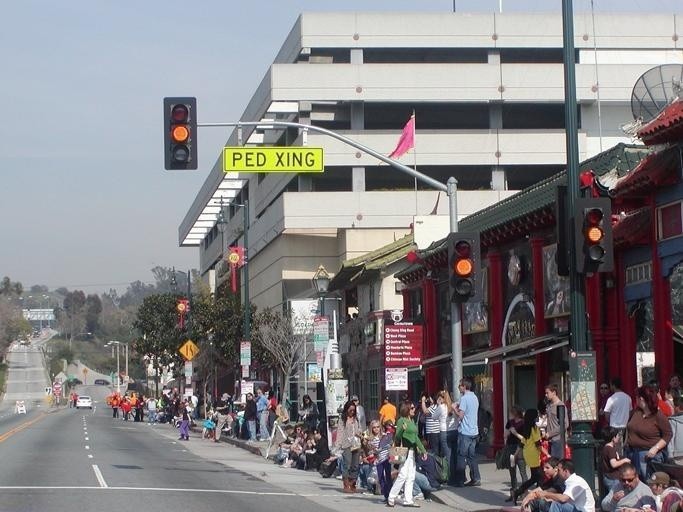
[385,326,421,366]
[223,146,323,173]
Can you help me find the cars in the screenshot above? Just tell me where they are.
[69,377,82,385]
[95,379,109,385]
[17,328,42,345]
[75,395,93,408]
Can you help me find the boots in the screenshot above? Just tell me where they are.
[344,479,357,493]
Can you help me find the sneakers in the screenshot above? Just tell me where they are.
[467,480,480,485]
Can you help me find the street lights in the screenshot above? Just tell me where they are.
[6,294,51,331]
[309,263,333,319]
[68,332,91,352]
[104,340,129,396]
[170,266,196,427]
[214,195,251,380]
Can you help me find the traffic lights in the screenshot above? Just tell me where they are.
[451,235,480,302]
[579,201,610,266]
[164,97,197,170]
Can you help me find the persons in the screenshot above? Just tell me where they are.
[69,393,74,408]
[333,379,481,507]
[505,375,683,512]
[112,391,200,441]
[73,392,79,407]
[202,385,330,470]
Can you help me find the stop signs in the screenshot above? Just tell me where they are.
[54,382,62,390]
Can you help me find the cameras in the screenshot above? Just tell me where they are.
[418,397,430,404]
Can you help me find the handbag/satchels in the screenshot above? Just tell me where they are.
[388,436,408,464]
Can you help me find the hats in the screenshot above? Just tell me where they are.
[647,472,670,484]
